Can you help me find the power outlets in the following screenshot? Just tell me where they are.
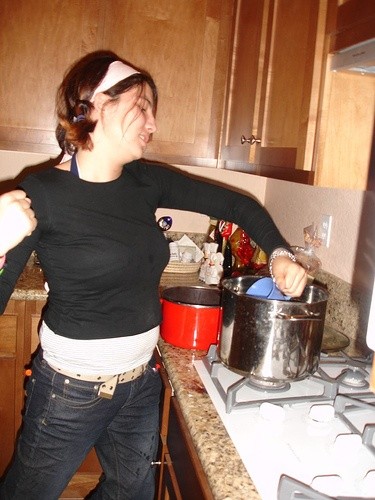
[319,214,332,248]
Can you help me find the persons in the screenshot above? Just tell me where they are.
[0,49,308,500]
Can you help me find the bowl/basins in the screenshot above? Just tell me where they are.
[322,326,350,352]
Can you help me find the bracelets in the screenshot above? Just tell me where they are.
[269,249,295,283]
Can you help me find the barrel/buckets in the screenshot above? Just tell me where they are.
[217,276,328,381]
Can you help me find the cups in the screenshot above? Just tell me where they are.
[246,278,291,301]
[169,243,196,262]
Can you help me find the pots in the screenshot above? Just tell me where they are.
[160,287,222,351]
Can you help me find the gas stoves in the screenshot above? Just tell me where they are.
[205,345,375,500]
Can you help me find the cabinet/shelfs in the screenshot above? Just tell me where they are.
[0,298,215,500]
[0,0,374,192]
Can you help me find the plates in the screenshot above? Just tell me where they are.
[164,262,199,273]
